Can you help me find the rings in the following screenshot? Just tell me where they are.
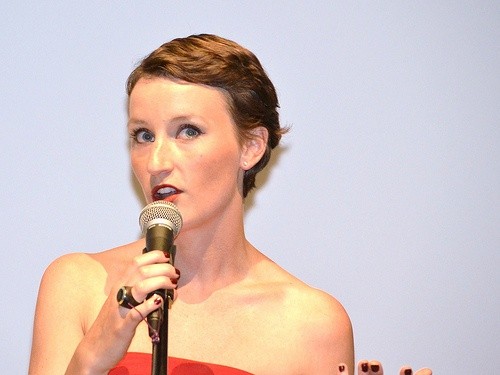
[116,285,142,307]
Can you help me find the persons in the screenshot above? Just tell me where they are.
[26,35,432,375]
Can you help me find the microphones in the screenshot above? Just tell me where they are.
[139,200,183,339]
